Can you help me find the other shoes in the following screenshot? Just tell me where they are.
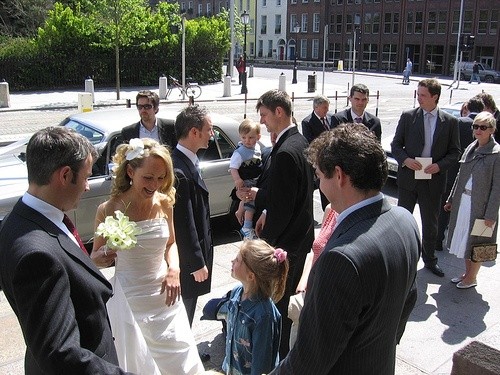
[456,280,477,288]
[425,263,445,278]
[240,226,258,239]
[199,352,210,362]
[451,276,466,282]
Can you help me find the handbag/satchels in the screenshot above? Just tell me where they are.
[470,226,497,262]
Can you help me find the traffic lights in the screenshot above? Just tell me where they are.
[467,35,475,49]
[456,33,465,50]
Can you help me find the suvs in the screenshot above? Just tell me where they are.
[452,60,500,84]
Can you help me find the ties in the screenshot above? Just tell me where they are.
[62,214,89,258]
[355,117,362,123]
[320,117,330,131]
[195,159,201,178]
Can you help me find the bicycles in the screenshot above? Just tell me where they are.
[164,75,202,99]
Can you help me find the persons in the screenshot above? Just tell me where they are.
[0,125,136,375]
[444,111,500,288]
[238,55,243,85]
[267,122,421,375]
[222,239,289,375]
[120,90,178,147]
[435,93,500,251]
[402,58,412,83]
[390,79,462,277]
[90,138,205,375]
[170,105,214,362]
[228,84,383,370]
[469,61,481,84]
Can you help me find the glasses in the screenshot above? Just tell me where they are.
[137,104,154,111]
[472,124,493,131]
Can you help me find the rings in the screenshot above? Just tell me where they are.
[249,193,251,195]
[245,196,248,199]
[250,198,252,201]
[105,251,108,257]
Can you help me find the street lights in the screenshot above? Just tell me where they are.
[291,22,301,84]
[240,9,250,94]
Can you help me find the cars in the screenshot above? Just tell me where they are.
[381,99,469,189]
[0,106,275,256]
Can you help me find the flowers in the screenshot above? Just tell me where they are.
[126,138,144,161]
[274,248,287,263]
[86,198,144,256]
